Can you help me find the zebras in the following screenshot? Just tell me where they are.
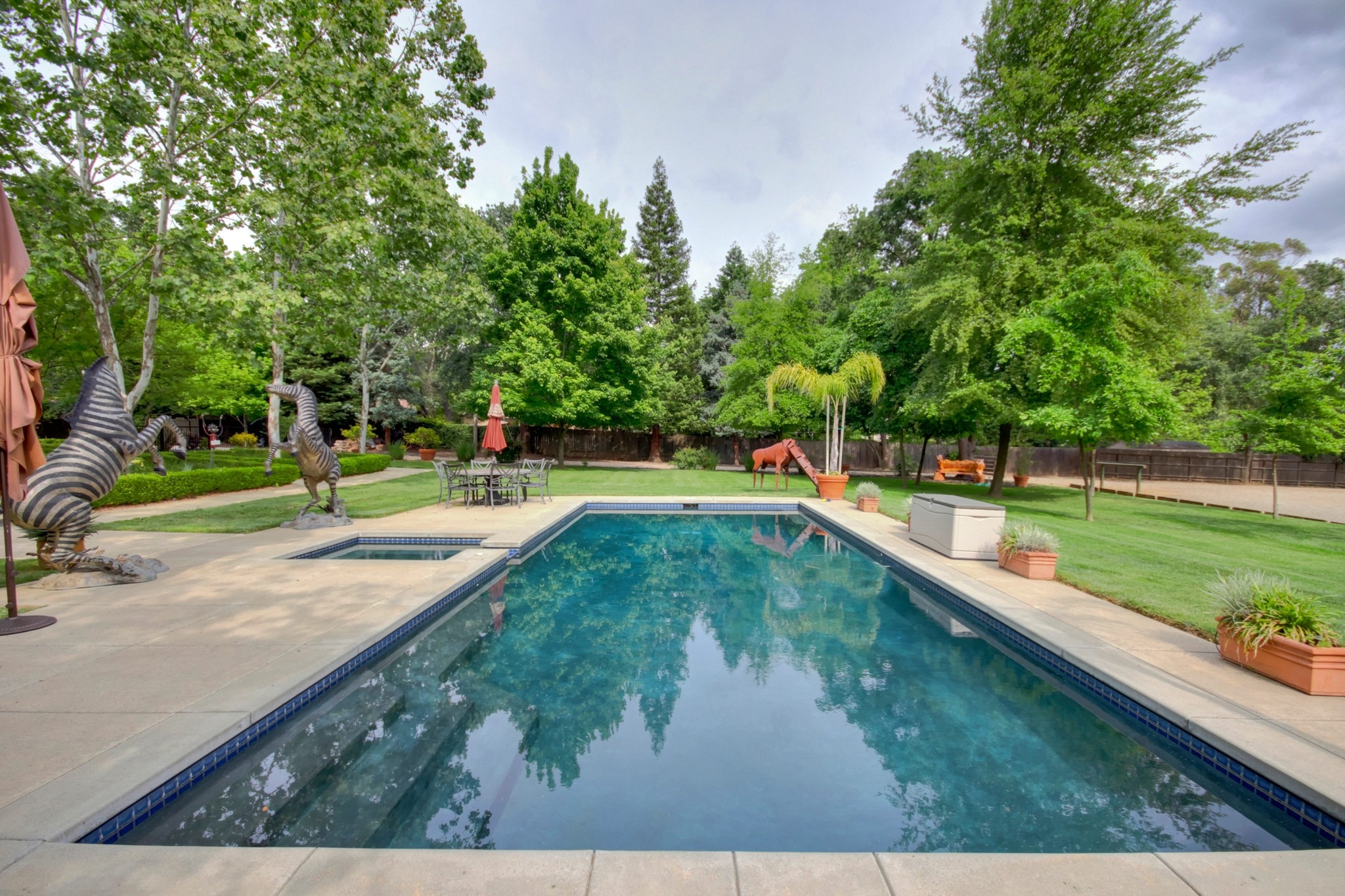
[6,353,188,575]
[264,379,342,519]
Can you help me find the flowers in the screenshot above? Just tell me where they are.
[902,495,917,515]
[817,470,848,476]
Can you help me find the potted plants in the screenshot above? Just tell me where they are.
[340,423,378,453]
[403,427,442,461]
[856,479,884,512]
[1198,565,1345,697]
[1013,447,1035,487]
[996,520,1059,580]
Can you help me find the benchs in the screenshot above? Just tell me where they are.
[911,493,1007,562]
[933,455,985,482]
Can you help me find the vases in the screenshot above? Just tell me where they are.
[817,475,849,500]
[908,513,911,531]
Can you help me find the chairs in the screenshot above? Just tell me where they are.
[430,458,555,510]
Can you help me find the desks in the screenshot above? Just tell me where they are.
[452,469,530,505]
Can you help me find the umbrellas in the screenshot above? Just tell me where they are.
[481,385,507,456]
[0,179,49,617]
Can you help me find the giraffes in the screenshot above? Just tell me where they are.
[752,438,821,496]
